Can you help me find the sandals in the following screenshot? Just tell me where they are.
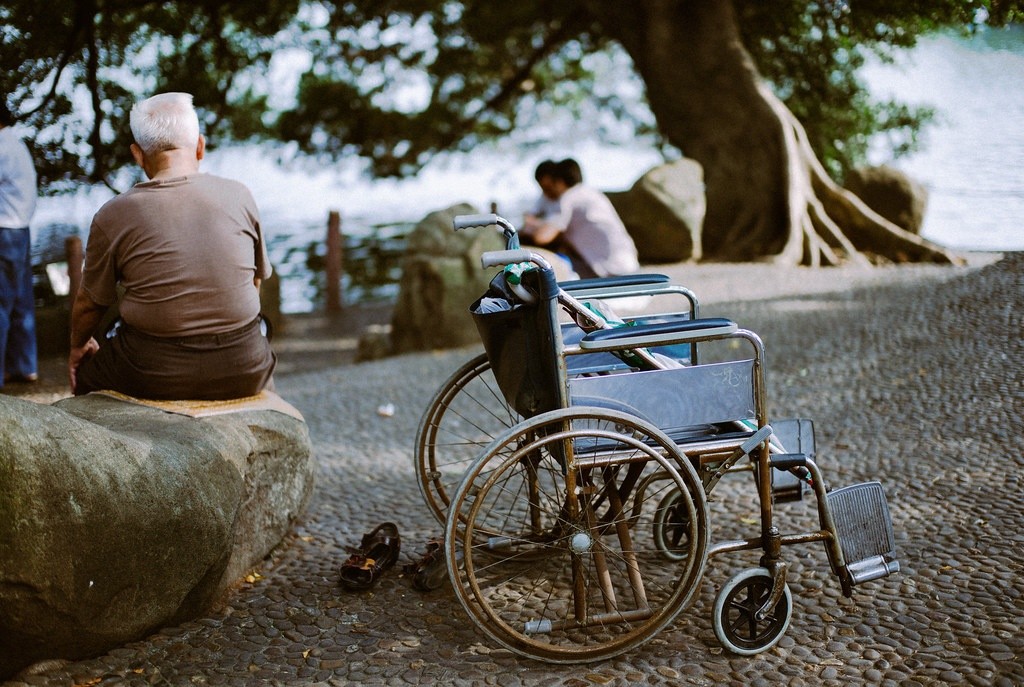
[339,522,401,590]
[413,536,465,591]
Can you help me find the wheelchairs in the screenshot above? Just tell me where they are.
[410,212,900,668]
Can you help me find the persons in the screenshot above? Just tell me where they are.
[0,102,38,392]
[69,92,276,402]
[514,159,640,280]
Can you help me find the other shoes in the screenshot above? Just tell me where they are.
[25,373,38,382]
